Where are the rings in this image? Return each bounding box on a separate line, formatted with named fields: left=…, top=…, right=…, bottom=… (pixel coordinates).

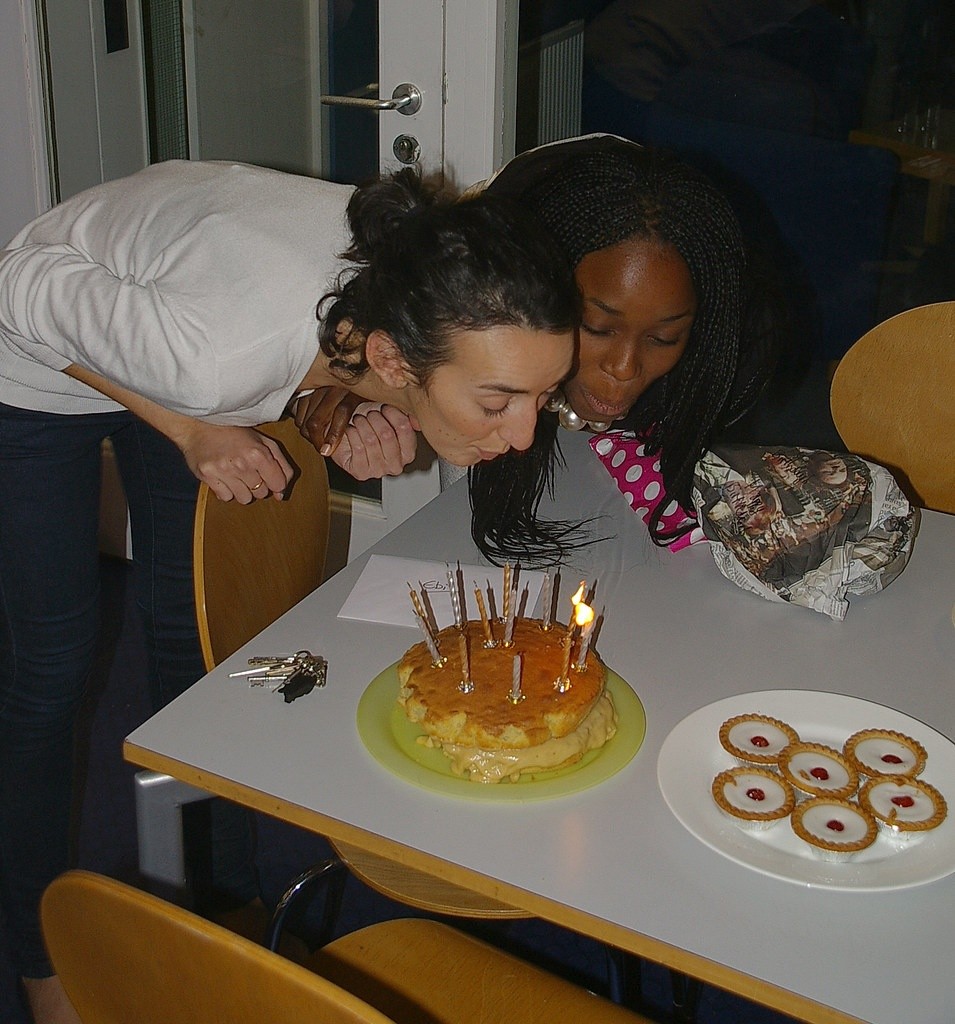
left=251, top=479, right=263, bottom=490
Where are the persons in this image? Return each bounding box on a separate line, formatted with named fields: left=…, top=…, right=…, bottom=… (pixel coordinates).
left=0, top=157, right=580, bottom=1023
left=294, top=133, right=747, bottom=570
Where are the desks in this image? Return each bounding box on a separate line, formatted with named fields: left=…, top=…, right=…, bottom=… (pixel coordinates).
left=848, top=107, right=955, bottom=246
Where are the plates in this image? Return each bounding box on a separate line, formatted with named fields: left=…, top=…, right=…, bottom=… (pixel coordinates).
left=355, top=659, right=646, bottom=802
left=656, top=689, right=955, bottom=890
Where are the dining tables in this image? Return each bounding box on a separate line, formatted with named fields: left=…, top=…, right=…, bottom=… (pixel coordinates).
left=117, top=424, right=954, bottom=1023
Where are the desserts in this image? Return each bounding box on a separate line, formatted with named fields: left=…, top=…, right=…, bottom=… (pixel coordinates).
left=710, top=712, right=947, bottom=862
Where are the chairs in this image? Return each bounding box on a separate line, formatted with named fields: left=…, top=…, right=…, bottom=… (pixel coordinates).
left=592, top=4, right=898, bottom=356
left=192, top=416, right=624, bottom=1006
left=828, top=302, right=955, bottom=515
left=40, top=869, right=657, bottom=1024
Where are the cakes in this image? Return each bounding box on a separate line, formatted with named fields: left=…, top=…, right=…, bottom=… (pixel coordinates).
left=397, top=616, right=619, bottom=783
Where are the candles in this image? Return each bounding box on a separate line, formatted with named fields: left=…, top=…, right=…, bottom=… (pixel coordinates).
left=406, top=562, right=606, bottom=705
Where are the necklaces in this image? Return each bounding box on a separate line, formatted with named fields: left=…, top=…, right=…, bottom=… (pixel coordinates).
left=545, top=389, right=628, bottom=431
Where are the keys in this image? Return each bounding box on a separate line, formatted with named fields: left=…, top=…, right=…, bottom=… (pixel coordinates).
left=230, top=650, right=328, bottom=704
